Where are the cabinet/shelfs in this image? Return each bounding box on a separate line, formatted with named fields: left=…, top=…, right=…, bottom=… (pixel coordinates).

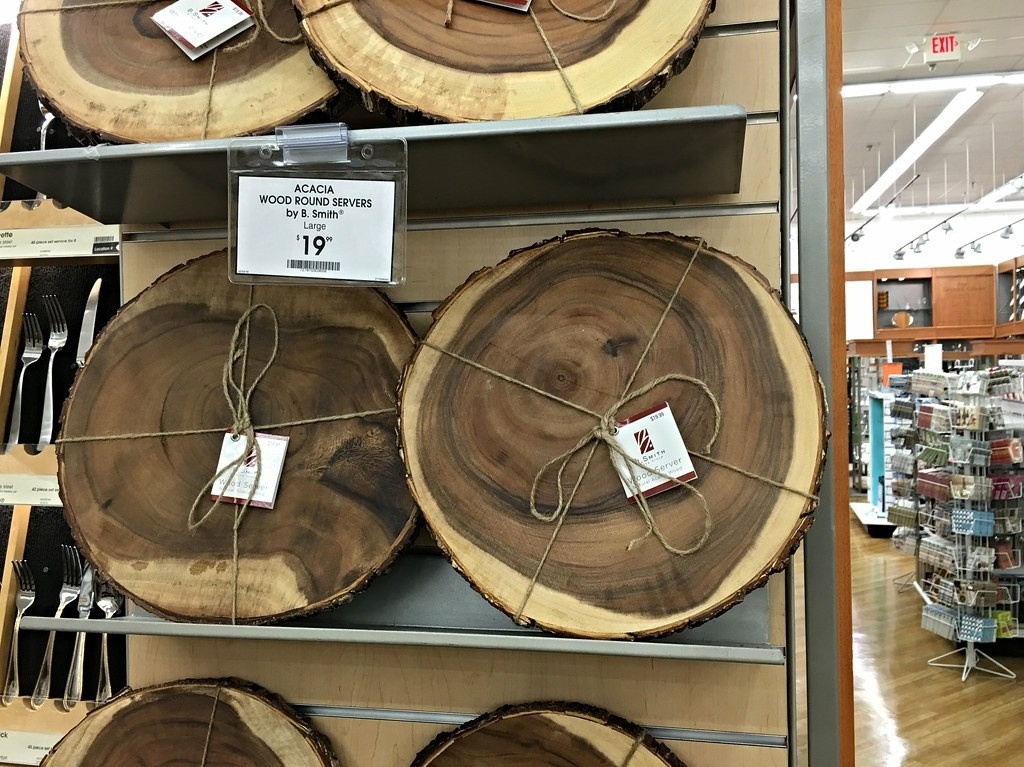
left=0, top=0, right=856, bottom=767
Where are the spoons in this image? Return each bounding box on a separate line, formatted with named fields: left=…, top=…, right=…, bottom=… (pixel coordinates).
left=94, top=573, right=124, bottom=708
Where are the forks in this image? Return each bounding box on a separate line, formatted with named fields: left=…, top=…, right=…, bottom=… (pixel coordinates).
left=35, top=295, right=68, bottom=452
left=2, top=559, right=36, bottom=706
left=4, top=312, right=43, bottom=455
left=30, top=544, right=83, bottom=710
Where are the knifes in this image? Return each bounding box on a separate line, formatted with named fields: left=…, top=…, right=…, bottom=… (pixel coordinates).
left=76, top=277, right=102, bottom=369
left=62, top=557, right=95, bottom=712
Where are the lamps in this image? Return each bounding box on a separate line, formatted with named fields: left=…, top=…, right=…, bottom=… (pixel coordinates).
left=850, top=221, right=1014, bottom=259
